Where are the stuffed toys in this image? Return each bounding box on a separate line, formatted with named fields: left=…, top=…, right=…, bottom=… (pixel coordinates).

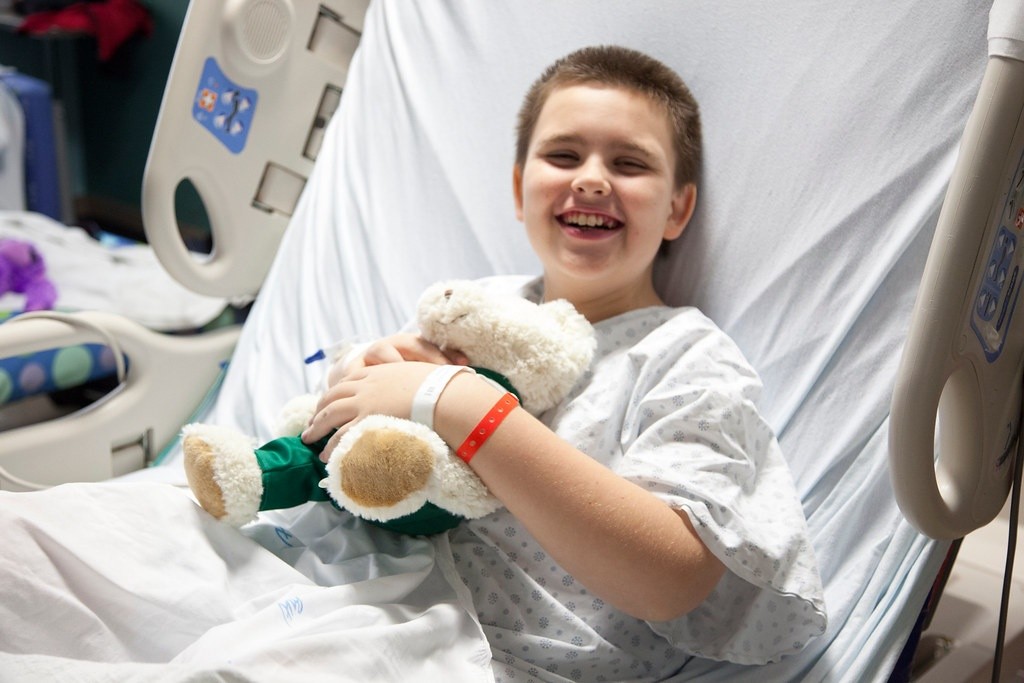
left=179, top=274, right=598, bottom=527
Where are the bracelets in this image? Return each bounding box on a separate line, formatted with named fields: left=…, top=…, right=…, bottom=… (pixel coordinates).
left=455, top=391, right=520, bottom=467
left=409, top=364, right=477, bottom=432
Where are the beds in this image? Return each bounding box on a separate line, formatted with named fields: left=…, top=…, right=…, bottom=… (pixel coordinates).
left=0, top=0, right=1024, bottom=683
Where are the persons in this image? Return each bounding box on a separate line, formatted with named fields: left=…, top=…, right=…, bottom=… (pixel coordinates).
left=300, top=44, right=828, bottom=683
